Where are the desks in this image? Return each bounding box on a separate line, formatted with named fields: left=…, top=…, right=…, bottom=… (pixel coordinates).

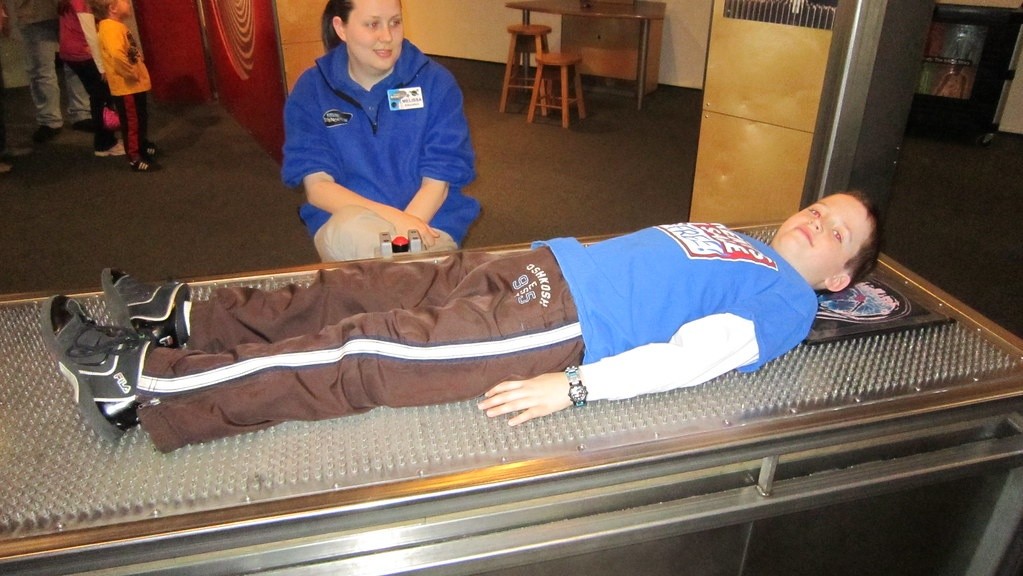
left=506, top=0, right=666, bottom=112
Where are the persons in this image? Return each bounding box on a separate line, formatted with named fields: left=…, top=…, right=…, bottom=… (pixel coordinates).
left=41, top=187, right=885, bottom=451
left=0, top=0, right=93, bottom=173
left=279, top=0, right=484, bottom=264
left=90, top=0, right=171, bottom=170
left=57, top=0, right=127, bottom=156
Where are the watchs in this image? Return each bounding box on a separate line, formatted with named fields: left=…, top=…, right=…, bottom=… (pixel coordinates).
left=563, top=366, right=589, bottom=408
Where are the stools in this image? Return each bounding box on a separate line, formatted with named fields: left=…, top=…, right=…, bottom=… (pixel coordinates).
left=527, top=53, right=586, bottom=128
left=500, top=25, right=552, bottom=116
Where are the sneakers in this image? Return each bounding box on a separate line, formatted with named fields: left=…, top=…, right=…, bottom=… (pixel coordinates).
left=41, top=294, right=156, bottom=441
left=94, top=140, right=126, bottom=156
left=102, top=266, right=190, bottom=348
left=141, top=143, right=167, bottom=157
left=130, top=158, right=161, bottom=172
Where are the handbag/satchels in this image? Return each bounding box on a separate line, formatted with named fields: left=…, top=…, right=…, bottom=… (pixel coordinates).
left=103, top=104, right=120, bottom=132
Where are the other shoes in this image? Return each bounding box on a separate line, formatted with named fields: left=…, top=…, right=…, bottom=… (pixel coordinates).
left=0, top=161, right=13, bottom=173
left=73, top=118, right=95, bottom=132
left=32, top=125, right=62, bottom=141
